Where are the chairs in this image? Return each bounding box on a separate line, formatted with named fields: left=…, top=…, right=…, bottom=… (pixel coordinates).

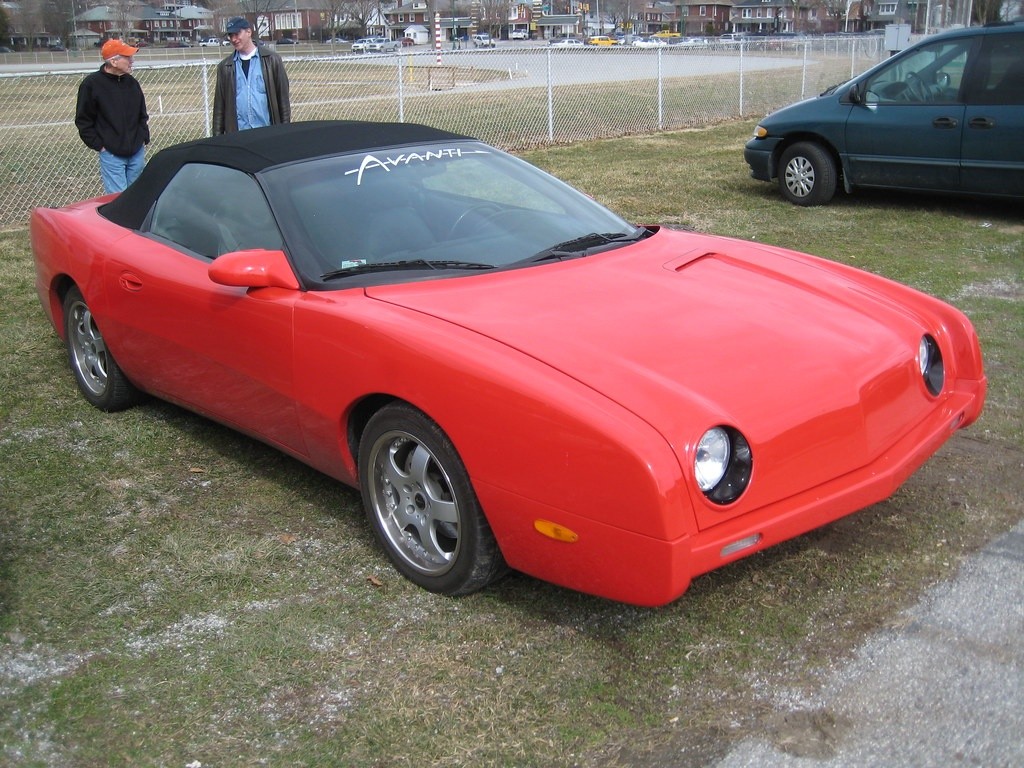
left=979, top=58, right=1024, bottom=104
left=219, top=192, right=285, bottom=255
left=360, top=175, right=437, bottom=262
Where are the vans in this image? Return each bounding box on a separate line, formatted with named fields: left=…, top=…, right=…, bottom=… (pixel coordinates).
left=717, top=33, right=740, bottom=44
left=473, top=35, right=478, bottom=42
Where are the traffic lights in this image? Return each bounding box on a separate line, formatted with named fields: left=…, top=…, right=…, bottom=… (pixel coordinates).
left=567, top=6, right=570, bottom=14
left=586, top=3, right=590, bottom=12
left=572, top=6, right=576, bottom=13
left=579, top=2, right=583, bottom=10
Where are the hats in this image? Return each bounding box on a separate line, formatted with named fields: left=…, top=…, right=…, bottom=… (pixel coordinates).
left=102, top=40, right=139, bottom=60
left=222, top=17, right=249, bottom=38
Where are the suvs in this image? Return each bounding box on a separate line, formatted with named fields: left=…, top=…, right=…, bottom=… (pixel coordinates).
left=512, top=28, right=529, bottom=40
left=589, top=35, right=619, bottom=47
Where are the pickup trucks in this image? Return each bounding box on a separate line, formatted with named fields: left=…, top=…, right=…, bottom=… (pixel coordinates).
left=551, top=39, right=585, bottom=50
left=654, top=30, right=682, bottom=38
left=367, top=38, right=397, bottom=52
left=198, top=38, right=228, bottom=48
left=548, top=33, right=584, bottom=42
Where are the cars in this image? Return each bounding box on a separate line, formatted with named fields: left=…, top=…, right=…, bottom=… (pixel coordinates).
left=130, top=41, right=149, bottom=47
left=168, top=41, right=193, bottom=48
left=867, top=29, right=887, bottom=36
left=632, top=38, right=667, bottom=47
left=254, top=39, right=266, bottom=48
left=222, top=39, right=230, bottom=46
left=327, top=37, right=347, bottom=45
left=368, top=37, right=376, bottom=43
left=276, top=38, right=299, bottom=45
left=397, top=37, right=413, bottom=46
left=745, top=23, right=1024, bottom=209
left=0, top=46, right=15, bottom=53
left=50, top=44, right=66, bottom=51
left=33, top=113, right=990, bottom=607
left=352, top=39, right=368, bottom=53
left=476, top=36, right=496, bottom=48
left=94, top=39, right=105, bottom=48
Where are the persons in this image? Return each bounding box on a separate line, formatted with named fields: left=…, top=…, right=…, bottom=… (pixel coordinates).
left=75, top=40, right=150, bottom=193
left=212, top=17, right=290, bottom=136
